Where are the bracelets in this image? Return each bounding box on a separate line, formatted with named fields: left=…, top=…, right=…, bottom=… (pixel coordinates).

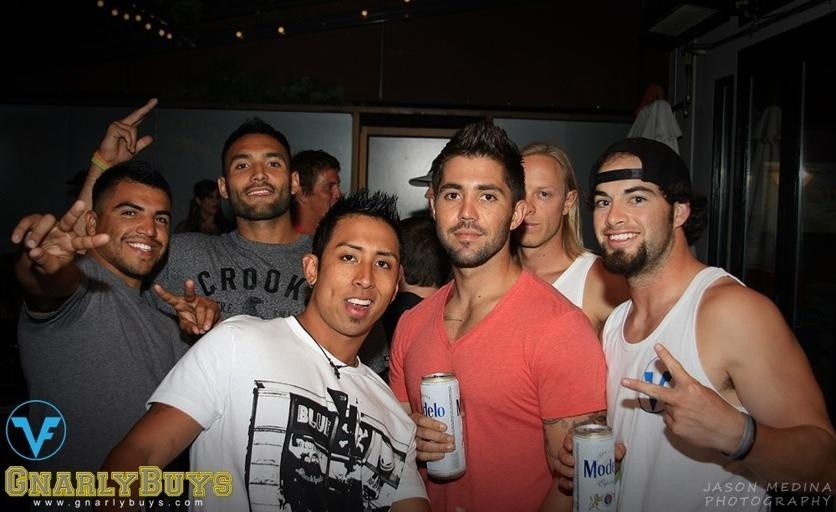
left=89, top=150, right=118, bottom=171
left=720, top=412, right=757, bottom=463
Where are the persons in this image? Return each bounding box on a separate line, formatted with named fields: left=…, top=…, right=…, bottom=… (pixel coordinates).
left=510, top=142, right=631, bottom=339
left=8, top=159, right=224, bottom=511
left=286, top=149, right=344, bottom=239
left=89, top=188, right=434, bottom=512
left=387, top=118, right=609, bottom=512
left=550, top=136, right=835, bottom=512
left=71, top=96, right=315, bottom=321
left=408, top=156, right=437, bottom=220
left=174, top=179, right=232, bottom=235
left=356, top=209, right=451, bottom=388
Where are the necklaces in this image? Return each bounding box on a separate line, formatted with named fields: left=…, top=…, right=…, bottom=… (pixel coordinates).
left=288, top=314, right=362, bottom=380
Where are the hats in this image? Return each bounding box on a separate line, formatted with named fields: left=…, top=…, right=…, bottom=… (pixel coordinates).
left=408, top=171, right=434, bottom=186
left=589, top=136, right=696, bottom=203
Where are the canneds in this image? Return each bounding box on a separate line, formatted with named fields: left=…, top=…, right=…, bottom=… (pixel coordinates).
left=573, top=425, right=616, bottom=512
left=419, top=373, right=467, bottom=483
